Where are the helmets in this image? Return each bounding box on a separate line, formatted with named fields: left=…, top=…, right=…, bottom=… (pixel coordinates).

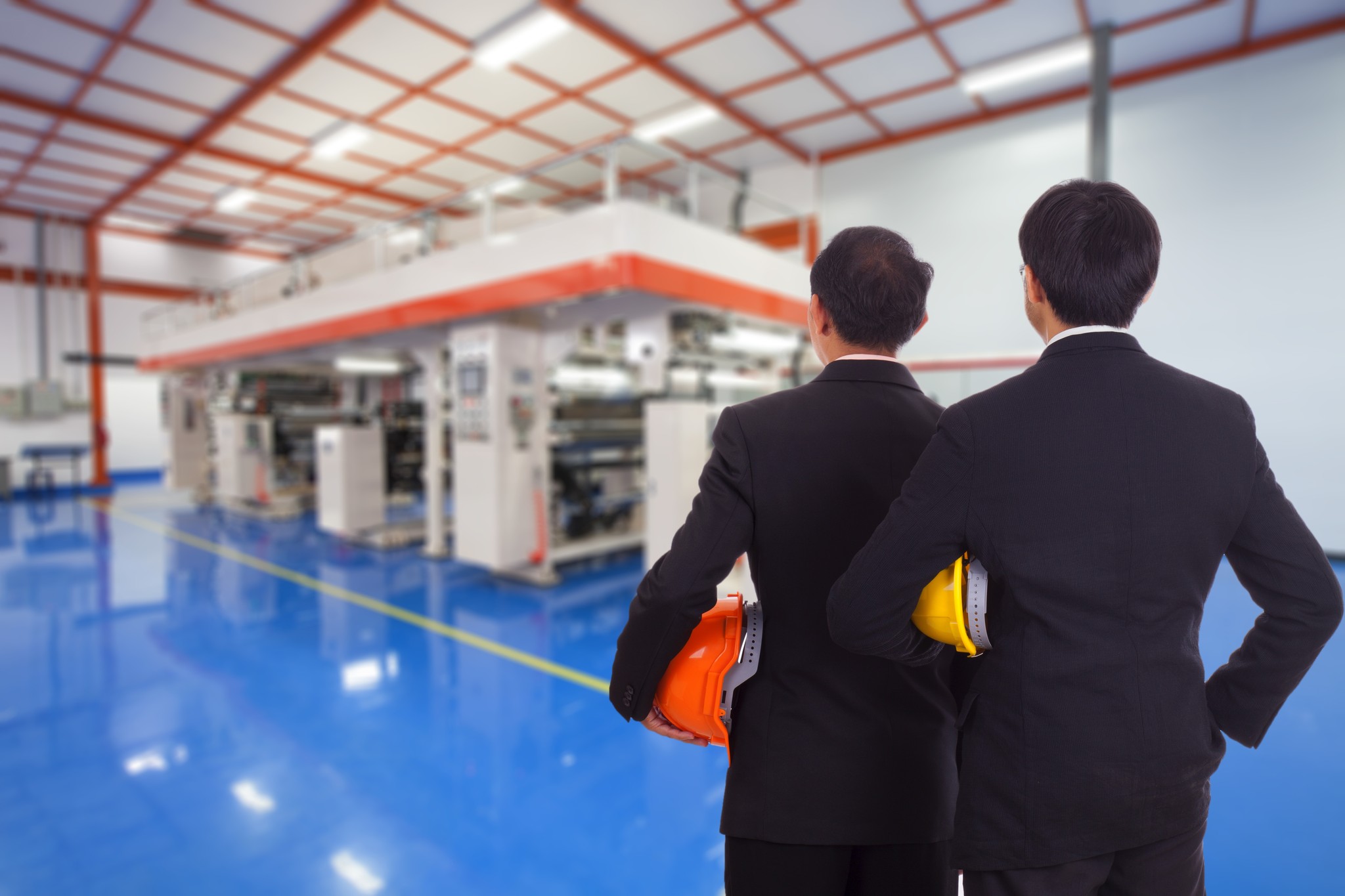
left=651, top=591, right=764, bottom=768
left=910, top=550, right=993, bottom=658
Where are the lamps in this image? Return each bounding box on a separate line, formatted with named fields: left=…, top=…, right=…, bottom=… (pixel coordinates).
left=469, top=4, right=570, bottom=74
left=629, top=103, right=715, bottom=145
left=961, top=30, right=1092, bottom=92
left=209, top=187, right=253, bottom=212
left=309, top=117, right=370, bottom=162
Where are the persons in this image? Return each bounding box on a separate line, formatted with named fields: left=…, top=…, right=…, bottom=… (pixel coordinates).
left=818, top=177, right=1345, bottom=896
left=607, top=223, right=963, bottom=896
